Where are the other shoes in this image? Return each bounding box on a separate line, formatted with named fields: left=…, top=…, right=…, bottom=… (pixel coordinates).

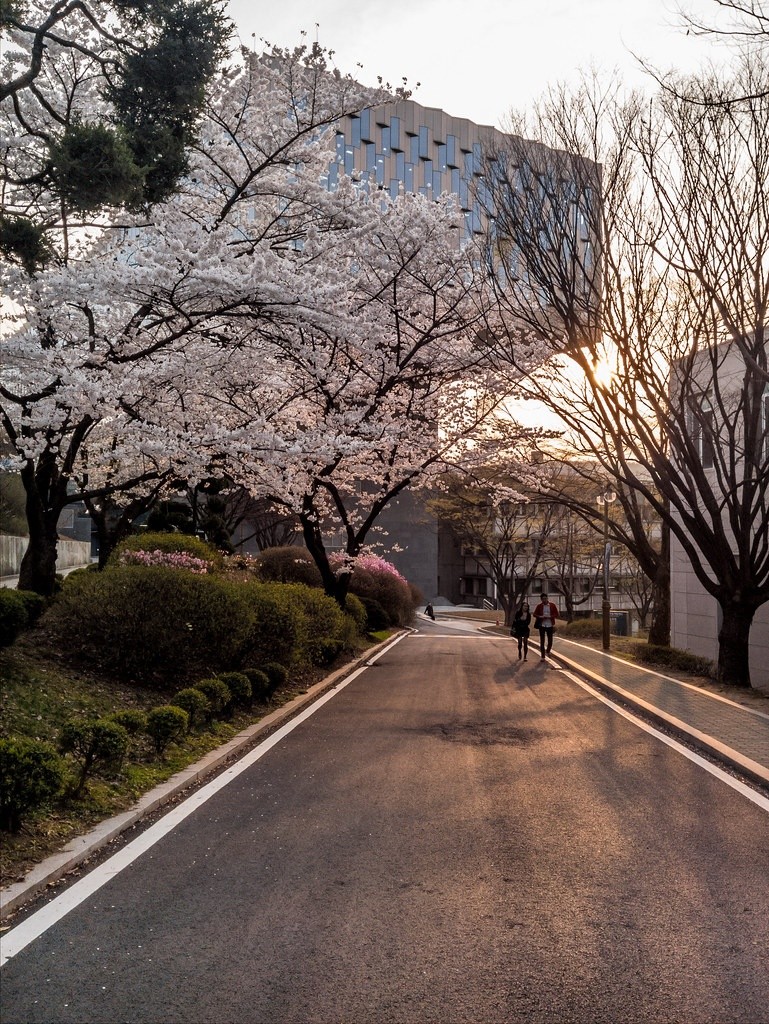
left=518, top=652, right=522, bottom=659
left=541, top=652, right=545, bottom=658
left=524, top=655, right=528, bottom=661
left=546, top=649, right=553, bottom=659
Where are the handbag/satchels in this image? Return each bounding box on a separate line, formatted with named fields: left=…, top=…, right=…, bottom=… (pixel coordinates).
left=510, top=611, right=521, bottom=638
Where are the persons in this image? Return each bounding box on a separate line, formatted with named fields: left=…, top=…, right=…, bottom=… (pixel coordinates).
left=514, top=602, right=531, bottom=661
left=424, top=601, right=435, bottom=620
left=533, top=593, right=558, bottom=662
